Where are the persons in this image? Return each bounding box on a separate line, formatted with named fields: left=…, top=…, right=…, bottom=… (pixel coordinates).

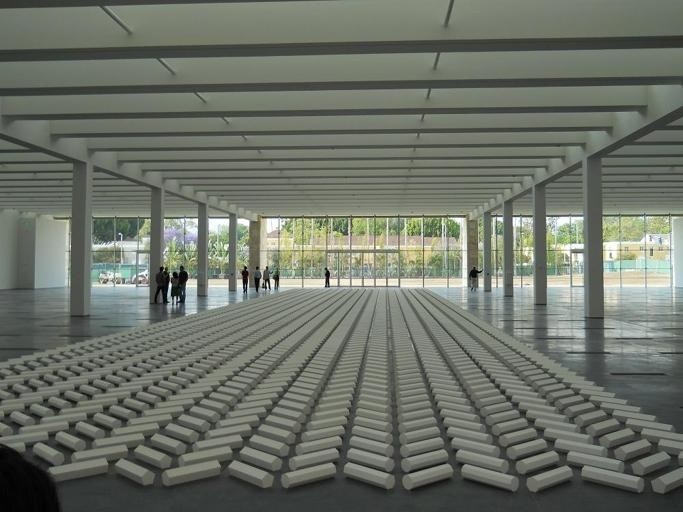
left=272, top=267, right=280, bottom=290
left=240, top=266, right=249, bottom=294
left=153, top=266, right=166, bottom=305
left=170, top=271, right=181, bottom=305
left=176, top=264, right=188, bottom=304
left=0, top=443, right=62, bottom=512
left=323, top=267, right=331, bottom=288
left=163, top=266, right=170, bottom=303
left=467, top=266, right=483, bottom=292
left=262, top=265, right=273, bottom=290
left=252, top=266, right=262, bottom=292
